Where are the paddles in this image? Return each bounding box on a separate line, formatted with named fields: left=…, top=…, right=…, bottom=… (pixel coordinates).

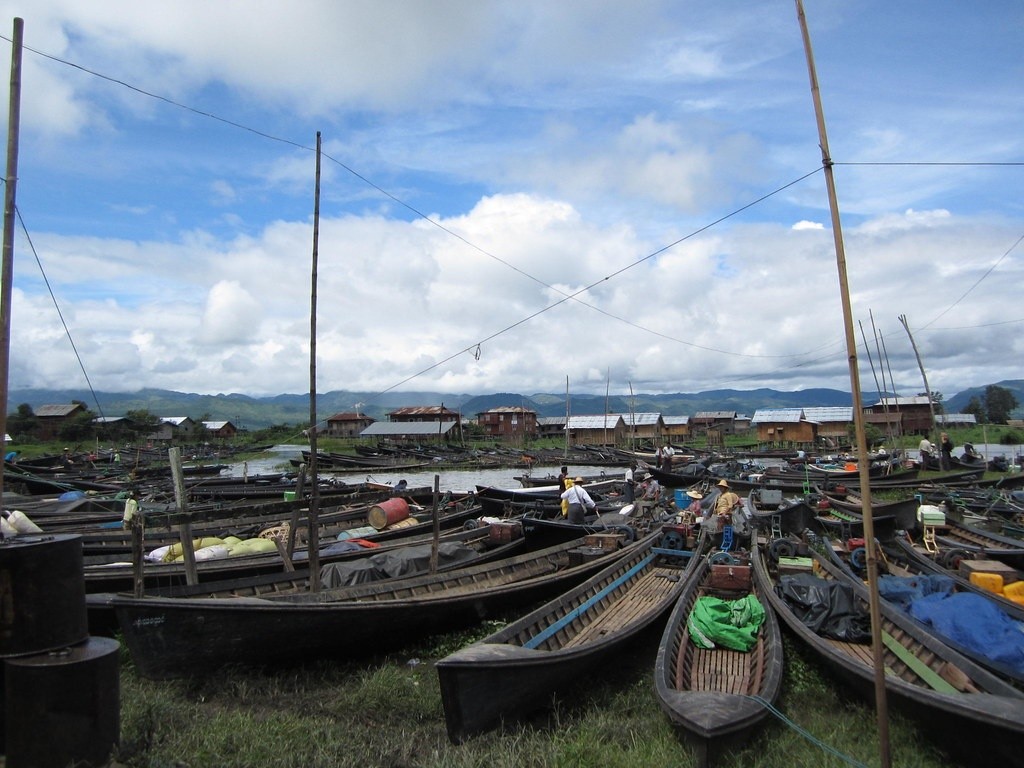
left=940, top=660, right=982, bottom=694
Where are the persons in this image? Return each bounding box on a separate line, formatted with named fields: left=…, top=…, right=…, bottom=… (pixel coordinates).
left=655, top=441, right=675, bottom=473
left=797, top=448, right=806, bottom=457
left=625, top=465, right=637, bottom=482
left=87, top=451, right=96, bottom=461
left=838, top=432, right=977, bottom=471
left=114, top=451, right=121, bottom=463
left=713, top=480, right=733, bottom=549
left=687, top=491, right=702, bottom=517
left=59, top=448, right=69, bottom=465
left=558, top=467, right=598, bottom=524
left=641, top=473, right=660, bottom=501
left=393, top=479, right=419, bottom=506
left=4, top=450, right=22, bottom=464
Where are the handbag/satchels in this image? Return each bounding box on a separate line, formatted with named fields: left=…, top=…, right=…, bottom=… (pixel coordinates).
left=581, top=503, right=587, bottom=514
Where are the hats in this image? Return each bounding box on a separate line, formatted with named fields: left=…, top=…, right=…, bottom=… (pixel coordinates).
left=715, top=480, right=730, bottom=488
left=643, top=473, right=654, bottom=480
left=686, top=490, right=702, bottom=498
left=573, top=477, right=583, bottom=483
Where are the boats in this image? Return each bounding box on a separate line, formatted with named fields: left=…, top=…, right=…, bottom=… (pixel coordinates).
left=3, top=437, right=1023, bottom=768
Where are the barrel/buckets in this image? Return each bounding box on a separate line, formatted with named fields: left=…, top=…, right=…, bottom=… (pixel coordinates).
left=0, top=636, right=122, bottom=768
left=337, top=498, right=419, bottom=541
left=0, top=531, right=89, bottom=658
left=674, top=489, right=692, bottom=510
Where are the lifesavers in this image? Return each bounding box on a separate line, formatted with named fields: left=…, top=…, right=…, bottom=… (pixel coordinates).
left=617, top=525, right=634, bottom=547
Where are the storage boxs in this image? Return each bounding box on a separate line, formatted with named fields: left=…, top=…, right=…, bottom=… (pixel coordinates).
left=748, top=473, right=764, bottom=483
left=778, top=555, right=812, bottom=580
left=490, top=522, right=521, bottom=546
left=712, top=564, right=752, bottom=589
left=585, top=533, right=625, bottom=553
left=760, top=489, right=781, bottom=504
left=921, top=511, right=945, bottom=525
left=958, top=560, right=1016, bottom=586
